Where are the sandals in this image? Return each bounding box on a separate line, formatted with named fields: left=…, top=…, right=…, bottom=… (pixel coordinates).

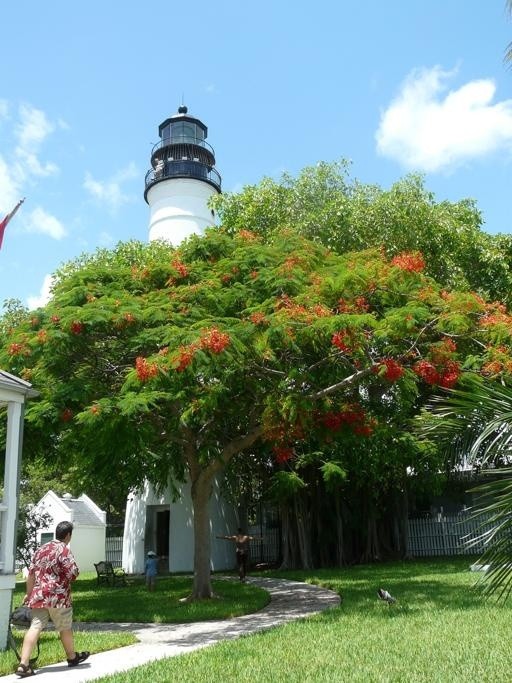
left=67, top=651, right=90, bottom=667
left=16, top=664, right=34, bottom=676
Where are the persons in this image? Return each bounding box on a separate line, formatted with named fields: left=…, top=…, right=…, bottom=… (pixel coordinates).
left=215, top=528, right=266, bottom=582
left=143, top=550, right=162, bottom=594
left=14, top=520, right=89, bottom=677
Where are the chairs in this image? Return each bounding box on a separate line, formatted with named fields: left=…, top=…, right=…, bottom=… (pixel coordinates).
left=93, top=561, right=128, bottom=586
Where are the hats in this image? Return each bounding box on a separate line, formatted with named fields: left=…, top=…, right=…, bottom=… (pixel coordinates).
left=148, top=551, right=156, bottom=556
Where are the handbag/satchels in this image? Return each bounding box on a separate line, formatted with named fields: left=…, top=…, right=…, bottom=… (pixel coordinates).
left=12, top=603, right=31, bottom=627
